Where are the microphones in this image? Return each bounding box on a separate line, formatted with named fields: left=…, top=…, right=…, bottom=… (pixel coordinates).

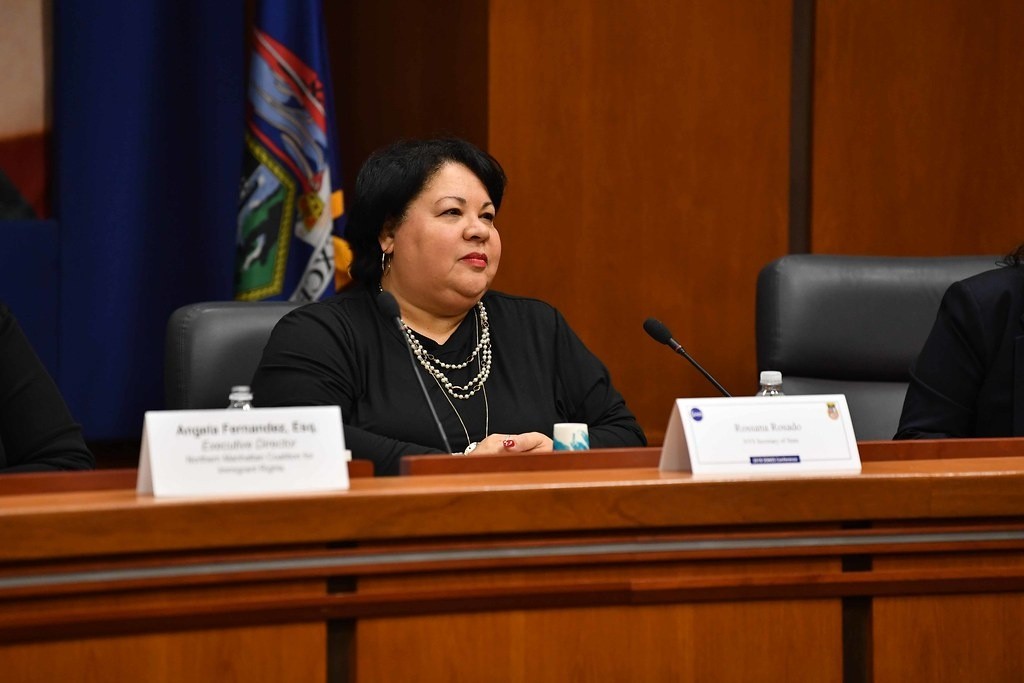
left=643, top=318, right=732, bottom=397
left=375, top=291, right=452, bottom=456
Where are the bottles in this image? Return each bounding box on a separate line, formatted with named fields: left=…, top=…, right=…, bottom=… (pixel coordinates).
left=756, top=370, right=787, bottom=396
left=226, top=385, right=254, bottom=409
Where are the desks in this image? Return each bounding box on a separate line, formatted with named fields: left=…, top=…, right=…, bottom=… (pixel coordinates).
left=0, top=438, right=1024, bottom=683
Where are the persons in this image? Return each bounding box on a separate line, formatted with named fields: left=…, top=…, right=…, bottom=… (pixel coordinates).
left=251, top=138, right=648, bottom=476
left=893, top=244, right=1024, bottom=439
left=0, top=302, right=97, bottom=474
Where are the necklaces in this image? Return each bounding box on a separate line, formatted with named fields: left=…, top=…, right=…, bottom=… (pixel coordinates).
left=396, top=300, right=492, bottom=452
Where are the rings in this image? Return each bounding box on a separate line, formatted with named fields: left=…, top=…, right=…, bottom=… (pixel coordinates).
left=505, top=435, right=509, bottom=440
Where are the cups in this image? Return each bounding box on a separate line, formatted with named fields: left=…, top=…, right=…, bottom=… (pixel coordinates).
left=553, top=422, right=589, bottom=451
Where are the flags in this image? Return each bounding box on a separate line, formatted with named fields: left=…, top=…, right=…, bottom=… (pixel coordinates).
left=53, top=0, right=353, bottom=441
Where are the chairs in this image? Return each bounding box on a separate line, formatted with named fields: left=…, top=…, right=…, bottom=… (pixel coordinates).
left=754, top=253, right=1022, bottom=440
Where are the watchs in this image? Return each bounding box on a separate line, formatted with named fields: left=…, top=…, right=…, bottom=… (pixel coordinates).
left=463, top=442, right=477, bottom=456
